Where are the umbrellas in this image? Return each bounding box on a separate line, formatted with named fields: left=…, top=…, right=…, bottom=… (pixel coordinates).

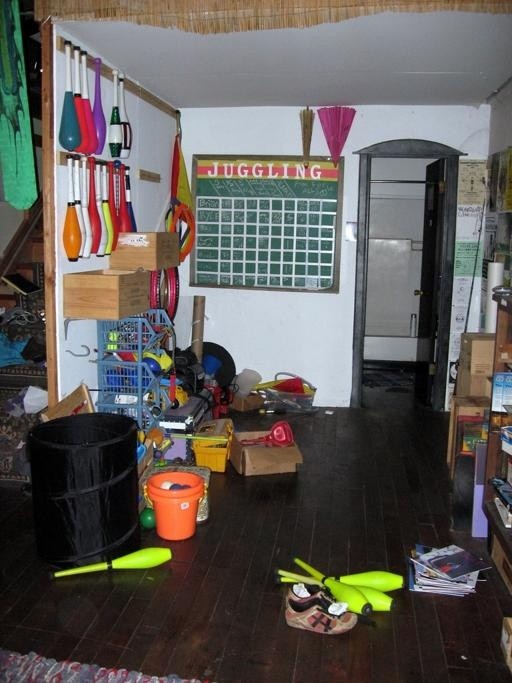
left=316, top=105, right=356, bottom=167
left=298, top=104, right=315, bottom=168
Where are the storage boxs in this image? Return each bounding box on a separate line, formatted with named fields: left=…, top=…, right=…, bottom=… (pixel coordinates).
left=499, top=615, right=512, bottom=674
left=453, top=364, right=493, bottom=399
left=459, top=332, right=496, bottom=373
left=193, top=417, right=234, bottom=472
left=109, top=230, right=182, bottom=272
left=264, top=387, right=317, bottom=413
left=229, top=392, right=264, bottom=412
left=63, top=268, right=151, bottom=323
left=230, top=429, right=304, bottom=476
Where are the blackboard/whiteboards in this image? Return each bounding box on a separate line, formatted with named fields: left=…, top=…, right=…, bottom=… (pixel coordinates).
left=189, top=151, right=346, bottom=296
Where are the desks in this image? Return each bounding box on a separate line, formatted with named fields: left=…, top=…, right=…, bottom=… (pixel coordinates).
left=446, top=392, right=491, bottom=480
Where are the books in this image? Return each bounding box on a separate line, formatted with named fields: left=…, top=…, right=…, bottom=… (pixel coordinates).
left=405, top=543, right=493, bottom=597
left=487, top=425, right=511, bottom=530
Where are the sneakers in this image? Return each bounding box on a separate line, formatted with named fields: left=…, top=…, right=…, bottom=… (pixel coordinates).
left=286, top=582, right=336, bottom=604
left=286, top=596, right=358, bottom=635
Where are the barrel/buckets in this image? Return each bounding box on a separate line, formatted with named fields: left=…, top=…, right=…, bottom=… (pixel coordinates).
left=142, top=468, right=210, bottom=544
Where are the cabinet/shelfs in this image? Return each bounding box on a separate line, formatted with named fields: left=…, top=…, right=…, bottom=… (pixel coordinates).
left=481, top=290, right=512, bottom=597
left=97, top=308, right=174, bottom=461
